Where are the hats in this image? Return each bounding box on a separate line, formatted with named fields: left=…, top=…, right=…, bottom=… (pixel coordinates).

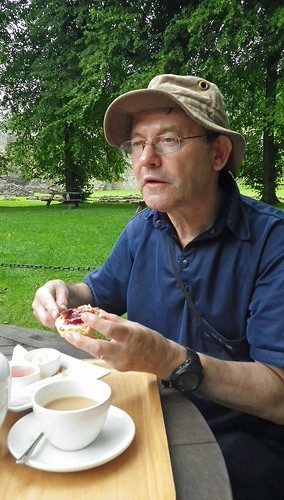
left=103, top=73, right=246, bottom=180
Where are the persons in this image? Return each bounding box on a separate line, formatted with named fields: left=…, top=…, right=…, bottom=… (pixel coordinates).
left=31, top=74, right=284, bottom=500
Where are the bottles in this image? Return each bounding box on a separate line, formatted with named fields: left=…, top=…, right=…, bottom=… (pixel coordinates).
left=0, top=353, right=10, bottom=425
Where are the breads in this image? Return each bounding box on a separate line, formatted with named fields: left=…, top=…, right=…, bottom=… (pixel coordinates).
left=55, top=304, right=105, bottom=338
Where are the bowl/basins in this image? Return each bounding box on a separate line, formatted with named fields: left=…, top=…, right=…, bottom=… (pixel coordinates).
left=28, top=348, right=61, bottom=378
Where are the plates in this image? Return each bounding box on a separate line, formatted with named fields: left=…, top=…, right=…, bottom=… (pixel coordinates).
left=7, top=405, right=136, bottom=472
left=8, top=348, right=111, bottom=412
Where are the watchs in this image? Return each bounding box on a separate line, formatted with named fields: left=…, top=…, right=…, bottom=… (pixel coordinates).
left=161, top=345, right=204, bottom=394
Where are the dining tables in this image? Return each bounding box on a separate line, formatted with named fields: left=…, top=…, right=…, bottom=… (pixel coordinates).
left=0, top=323, right=234, bottom=500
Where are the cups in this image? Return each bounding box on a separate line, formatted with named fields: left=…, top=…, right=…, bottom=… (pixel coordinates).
left=33, top=379, right=111, bottom=451
left=9, top=361, right=40, bottom=405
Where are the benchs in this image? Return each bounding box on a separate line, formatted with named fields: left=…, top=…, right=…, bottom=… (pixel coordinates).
left=131, top=200, right=146, bottom=214
left=42, top=191, right=82, bottom=209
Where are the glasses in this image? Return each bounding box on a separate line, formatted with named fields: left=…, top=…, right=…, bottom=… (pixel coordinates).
left=120, top=133, right=209, bottom=158
